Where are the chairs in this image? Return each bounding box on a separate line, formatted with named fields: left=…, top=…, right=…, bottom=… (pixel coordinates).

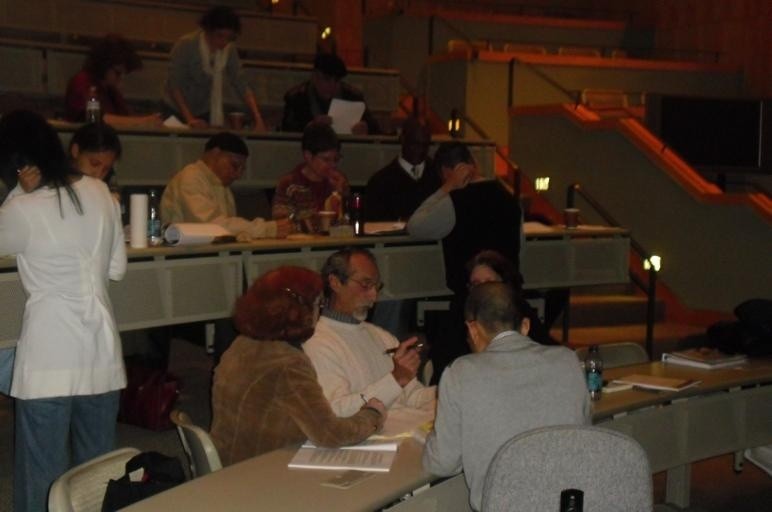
left=481, top=423, right=654, bottom=512
left=169, top=408, right=223, bottom=480
left=575, top=343, right=649, bottom=368
left=48, top=446, right=144, bottom=512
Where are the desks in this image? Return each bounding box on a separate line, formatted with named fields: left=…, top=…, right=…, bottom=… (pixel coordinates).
left=0, top=224, right=631, bottom=345
left=44, top=119, right=498, bottom=187
left=115, top=356, right=772, bottom=512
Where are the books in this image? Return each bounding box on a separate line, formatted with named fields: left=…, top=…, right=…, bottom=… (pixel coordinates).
left=612, top=373, right=701, bottom=391
left=288, top=439, right=403, bottom=473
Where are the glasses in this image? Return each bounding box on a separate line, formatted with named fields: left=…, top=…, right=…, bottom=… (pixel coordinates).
left=349, top=276, right=385, bottom=292
left=311, top=153, right=346, bottom=165
left=465, top=280, right=481, bottom=289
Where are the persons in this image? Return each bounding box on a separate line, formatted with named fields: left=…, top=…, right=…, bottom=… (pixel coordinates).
left=0, top=109, right=130, bottom=507
left=165, top=6, right=266, bottom=131
left=211, top=265, right=389, bottom=467
left=422, top=278, right=596, bottom=512
left=155, top=131, right=298, bottom=241
left=449, top=245, right=561, bottom=368
left=363, top=112, right=442, bottom=226
left=284, top=54, right=379, bottom=135
left=407, top=141, right=525, bottom=350
left=301, top=246, right=443, bottom=422
left=64, top=35, right=167, bottom=131
left=273, top=123, right=352, bottom=228
left=65, top=121, right=124, bottom=182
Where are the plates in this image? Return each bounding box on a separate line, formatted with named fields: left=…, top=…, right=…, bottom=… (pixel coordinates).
left=364, top=221, right=407, bottom=235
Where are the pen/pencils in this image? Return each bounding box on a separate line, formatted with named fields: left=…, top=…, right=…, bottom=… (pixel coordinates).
left=382, top=343, right=423, bottom=354
left=360, top=394, right=370, bottom=404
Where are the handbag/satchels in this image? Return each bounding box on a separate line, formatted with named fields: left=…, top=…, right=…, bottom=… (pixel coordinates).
left=115, top=356, right=182, bottom=431
left=101, top=449, right=189, bottom=512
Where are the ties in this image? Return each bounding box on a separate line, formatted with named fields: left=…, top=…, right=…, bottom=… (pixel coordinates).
left=412, top=164, right=421, bottom=179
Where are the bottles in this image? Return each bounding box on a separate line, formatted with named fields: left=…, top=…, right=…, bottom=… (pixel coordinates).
left=585, top=343, right=603, bottom=402
left=350, top=192, right=364, bottom=236
left=559, top=489, right=582, bottom=512
left=149, top=190, right=163, bottom=246
left=85, top=85, right=100, bottom=126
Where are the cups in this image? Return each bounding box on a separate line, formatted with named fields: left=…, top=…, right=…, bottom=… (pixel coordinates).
left=318, top=211, right=336, bottom=235
left=564, top=208, right=580, bottom=230
